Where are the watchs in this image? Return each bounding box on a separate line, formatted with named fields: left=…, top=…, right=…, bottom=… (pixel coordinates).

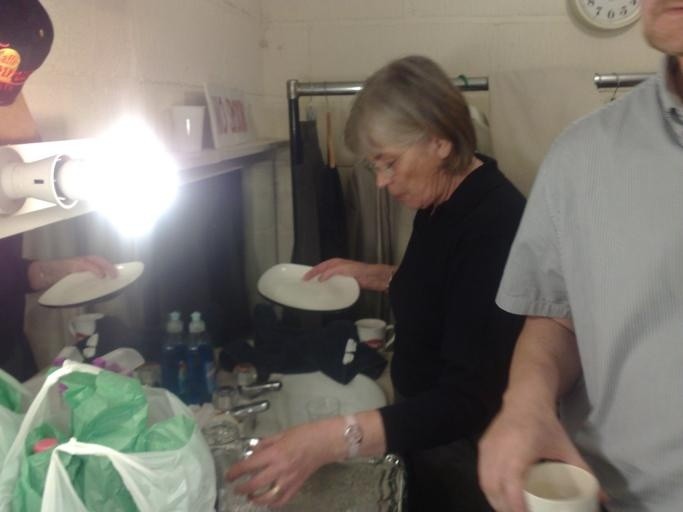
left=343, top=414, right=363, bottom=460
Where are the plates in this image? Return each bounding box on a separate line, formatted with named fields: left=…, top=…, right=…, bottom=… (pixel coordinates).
left=272, top=369, right=388, bottom=441
left=254, top=260, right=363, bottom=314
left=34, top=259, right=146, bottom=310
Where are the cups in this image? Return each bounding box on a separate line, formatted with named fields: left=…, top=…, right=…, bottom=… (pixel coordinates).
left=519, top=461, right=602, bottom=512
left=353, top=317, right=396, bottom=354
left=66, top=312, right=105, bottom=344
left=303, top=395, right=342, bottom=421
left=170, top=101, right=207, bottom=156
left=219, top=434, right=274, bottom=512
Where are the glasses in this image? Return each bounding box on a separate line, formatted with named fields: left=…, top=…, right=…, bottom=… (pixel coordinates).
left=360, top=149, right=414, bottom=179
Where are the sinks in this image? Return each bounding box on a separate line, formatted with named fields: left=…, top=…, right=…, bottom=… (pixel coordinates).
left=248, top=365, right=388, bottom=447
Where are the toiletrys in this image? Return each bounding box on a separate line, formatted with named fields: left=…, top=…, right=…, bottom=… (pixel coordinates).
left=160, top=310, right=214, bottom=405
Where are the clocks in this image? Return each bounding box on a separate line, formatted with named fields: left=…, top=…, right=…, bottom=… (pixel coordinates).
left=571, top=0, right=641, bottom=30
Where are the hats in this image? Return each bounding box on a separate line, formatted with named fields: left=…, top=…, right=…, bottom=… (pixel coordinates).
left=0, top=1, right=53, bottom=108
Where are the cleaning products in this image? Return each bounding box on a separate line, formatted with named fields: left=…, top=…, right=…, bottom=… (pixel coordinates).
left=160, top=311, right=185, bottom=403
left=186, top=309, right=217, bottom=403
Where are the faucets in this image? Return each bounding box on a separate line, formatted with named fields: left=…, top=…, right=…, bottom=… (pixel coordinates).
left=216, top=399, right=270, bottom=422
left=207, top=380, right=284, bottom=413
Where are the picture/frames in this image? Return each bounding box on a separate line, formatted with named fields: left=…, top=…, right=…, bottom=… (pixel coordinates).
left=200, top=84, right=254, bottom=146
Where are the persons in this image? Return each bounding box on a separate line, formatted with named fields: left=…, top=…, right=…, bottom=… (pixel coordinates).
left=0, top=234, right=118, bottom=382
left=219, top=63, right=527, bottom=512
left=476, top=0, right=683, bottom=512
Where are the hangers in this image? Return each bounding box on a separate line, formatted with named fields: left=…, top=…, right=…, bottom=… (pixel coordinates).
left=610, top=71, right=621, bottom=102
left=301, top=79, right=336, bottom=173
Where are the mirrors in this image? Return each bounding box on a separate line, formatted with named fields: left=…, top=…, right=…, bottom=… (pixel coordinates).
left=1, top=161, right=276, bottom=467
